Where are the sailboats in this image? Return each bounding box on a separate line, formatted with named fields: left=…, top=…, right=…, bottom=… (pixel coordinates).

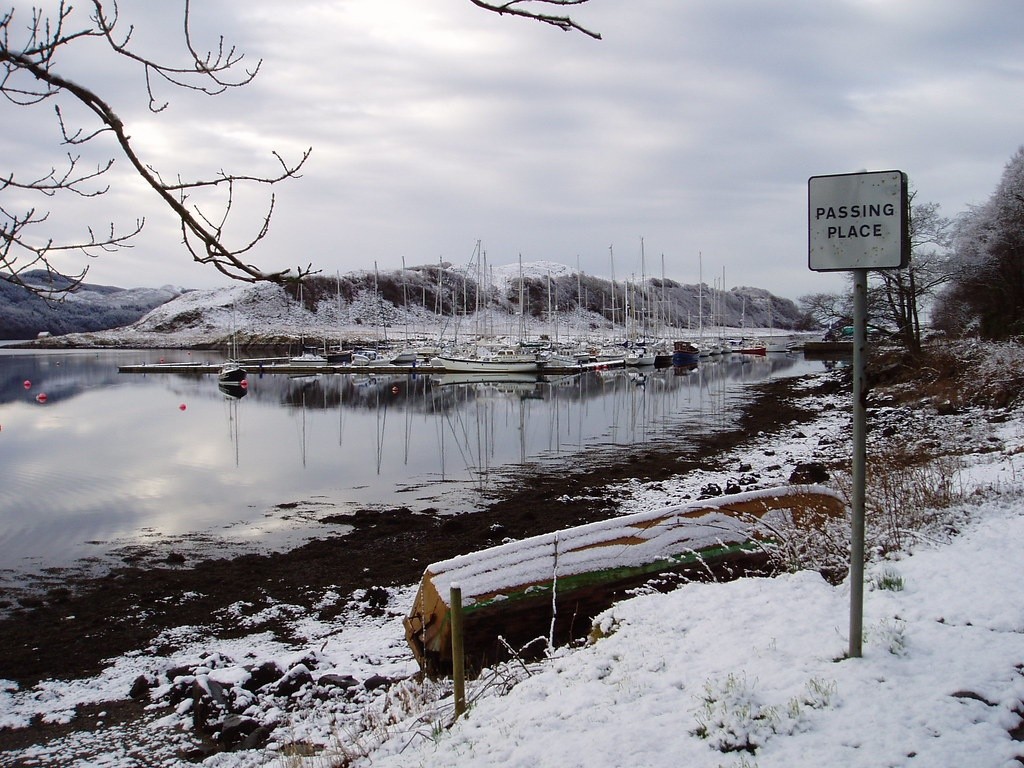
left=218, top=299, right=248, bottom=383
left=287, top=353, right=766, bottom=500
left=218, top=381, right=248, bottom=468
left=288, top=238, right=797, bottom=374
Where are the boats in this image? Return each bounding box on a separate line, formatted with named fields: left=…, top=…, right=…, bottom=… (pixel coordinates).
left=402, top=484, right=850, bottom=683
